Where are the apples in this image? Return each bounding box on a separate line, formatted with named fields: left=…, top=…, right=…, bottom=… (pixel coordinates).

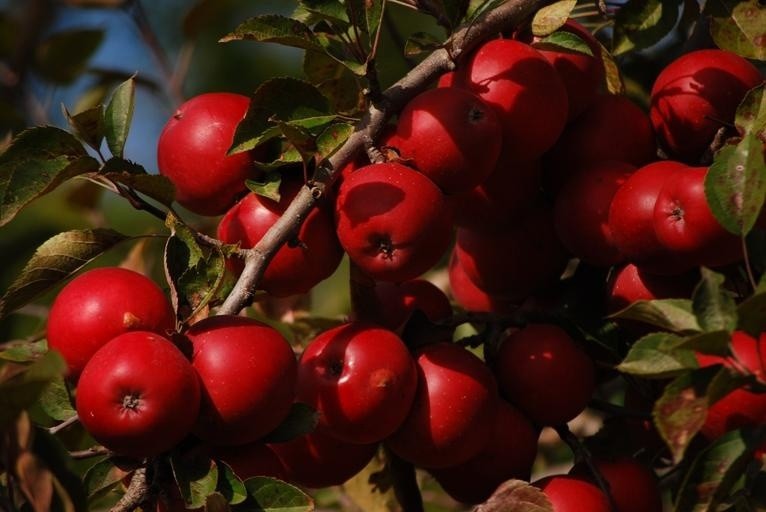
left=46, top=17, right=766, bottom=511
